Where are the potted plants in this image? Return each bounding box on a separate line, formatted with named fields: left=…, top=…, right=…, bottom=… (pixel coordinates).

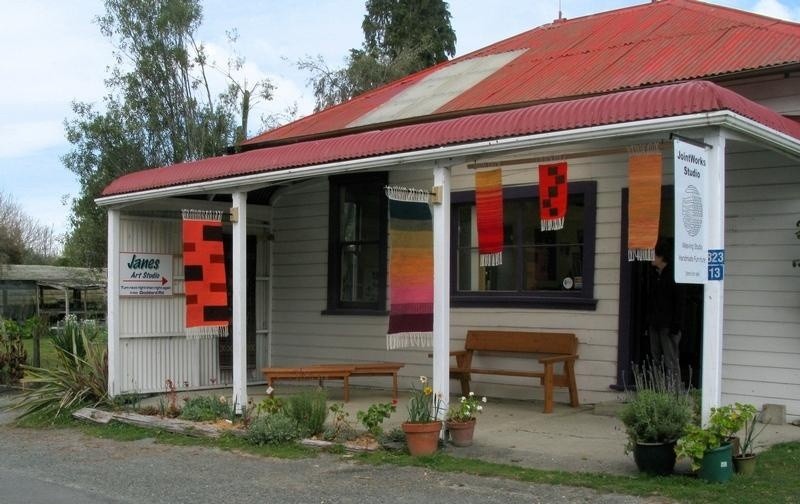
left=612, top=384, right=691, bottom=474
left=680, top=403, right=761, bottom=482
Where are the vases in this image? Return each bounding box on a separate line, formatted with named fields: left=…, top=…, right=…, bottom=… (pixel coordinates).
left=732, top=452, right=758, bottom=476
left=721, top=434, right=742, bottom=455
left=446, top=420, right=476, bottom=446
left=401, top=422, right=443, bottom=455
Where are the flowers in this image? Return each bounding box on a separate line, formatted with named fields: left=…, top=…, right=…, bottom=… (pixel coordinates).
left=454, top=388, right=491, bottom=420
left=403, top=375, right=445, bottom=422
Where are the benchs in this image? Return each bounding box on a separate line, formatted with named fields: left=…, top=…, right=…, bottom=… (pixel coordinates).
left=261, top=361, right=353, bottom=406
left=424, top=326, right=585, bottom=410
left=314, top=359, right=405, bottom=404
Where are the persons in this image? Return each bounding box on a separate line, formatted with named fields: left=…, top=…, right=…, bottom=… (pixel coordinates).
left=635, top=239, right=688, bottom=389
